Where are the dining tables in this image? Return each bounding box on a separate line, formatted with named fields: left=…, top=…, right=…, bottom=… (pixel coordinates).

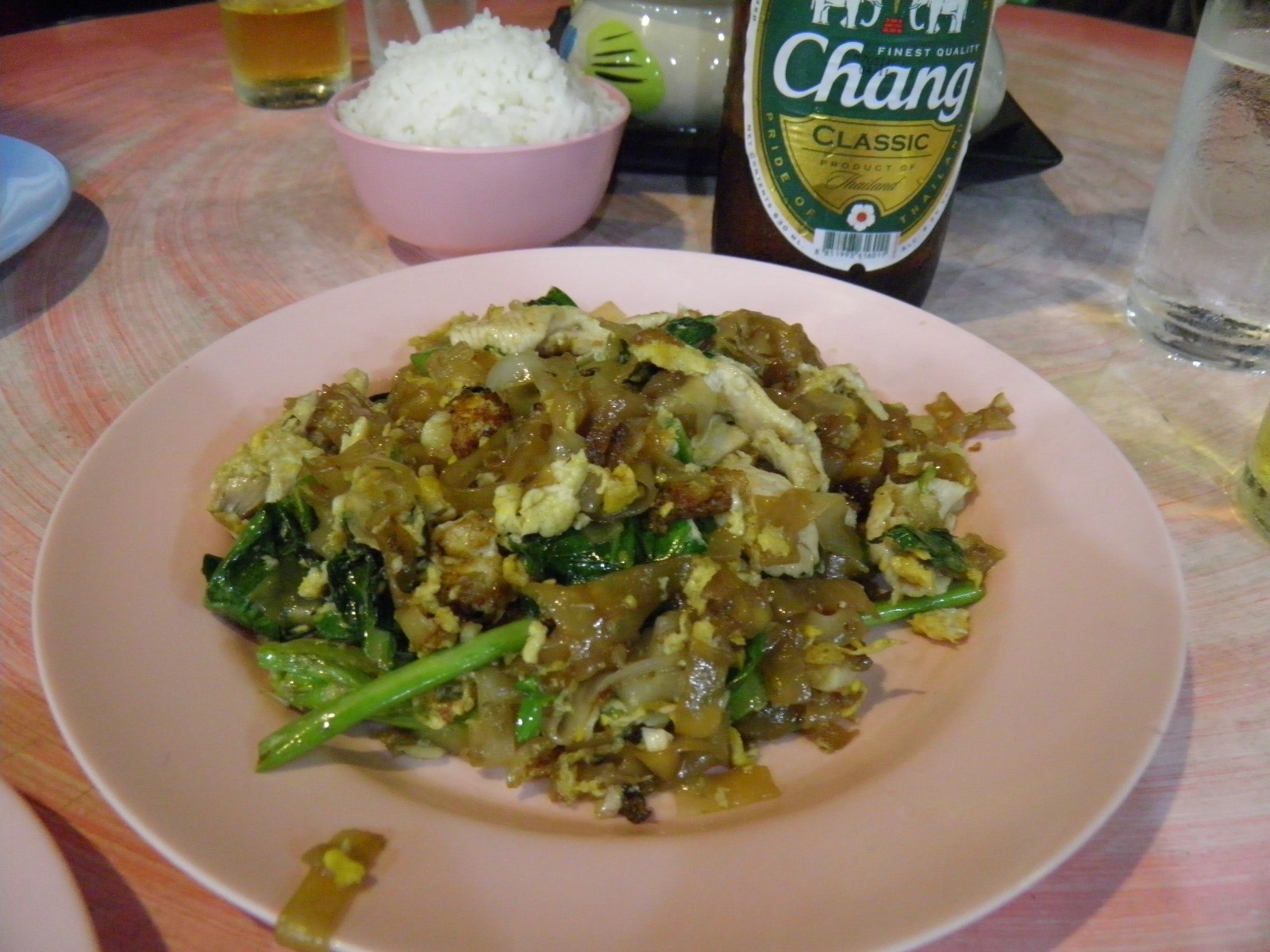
left=1, top=2, right=1266, bottom=951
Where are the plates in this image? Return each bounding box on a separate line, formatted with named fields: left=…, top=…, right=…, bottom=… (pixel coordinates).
left=34, top=248, right=1187, bottom=952
left=2, top=777, right=102, bottom=949
left=541, top=6, right=1063, bottom=182
left=0, top=134, right=71, bottom=264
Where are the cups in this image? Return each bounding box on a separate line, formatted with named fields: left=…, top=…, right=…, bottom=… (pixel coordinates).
left=1236, top=395, right=1269, bottom=540
left=217, top=0, right=351, bottom=108
left=363, top=0, right=476, bottom=73
left=971, top=1, right=1007, bottom=131
left=557, top=0, right=738, bottom=130
left=1128, top=0, right=1269, bottom=376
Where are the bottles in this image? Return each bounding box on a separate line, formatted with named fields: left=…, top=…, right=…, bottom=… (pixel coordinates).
left=712, top=1, right=993, bottom=308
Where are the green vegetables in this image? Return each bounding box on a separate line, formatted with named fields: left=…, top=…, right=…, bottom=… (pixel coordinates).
left=203, top=494, right=989, bottom=771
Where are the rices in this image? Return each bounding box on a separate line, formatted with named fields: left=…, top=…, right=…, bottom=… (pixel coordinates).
left=337, top=2, right=625, bottom=150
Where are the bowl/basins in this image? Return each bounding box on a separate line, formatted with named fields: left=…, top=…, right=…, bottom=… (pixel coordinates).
left=328, top=75, right=632, bottom=264
